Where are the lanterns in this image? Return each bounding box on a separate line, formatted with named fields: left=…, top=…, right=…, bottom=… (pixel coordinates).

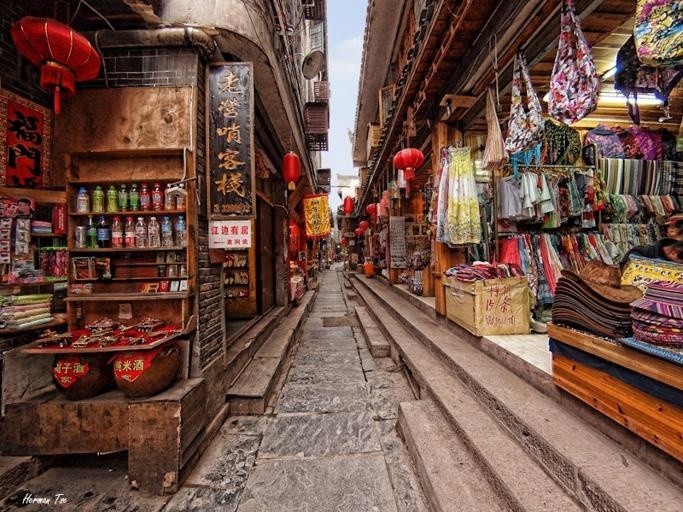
left=353, top=227, right=363, bottom=236
left=359, top=220, right=369, bottom=230
left=282, top=152, right=300, bottom=190
left=366, top=203, right=376, bottom=217
left=11, top=14, right=100, bottom=117
left=342, top=196, right=353, bottom=215
left=392, top=147, right=425, bottom=180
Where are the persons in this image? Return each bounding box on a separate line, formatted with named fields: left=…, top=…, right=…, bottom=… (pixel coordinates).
left=16, top=198, right=35, bottom=219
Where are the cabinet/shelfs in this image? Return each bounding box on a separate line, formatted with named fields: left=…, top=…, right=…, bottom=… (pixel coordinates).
left=63, top=211, right=187, bottom=301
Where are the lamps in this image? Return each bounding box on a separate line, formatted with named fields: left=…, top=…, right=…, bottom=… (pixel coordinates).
left=279, top=49, right=324, bottom=80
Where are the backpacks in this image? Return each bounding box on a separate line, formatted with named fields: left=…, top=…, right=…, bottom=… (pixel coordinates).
left=614, top=0, right=683, bottom=125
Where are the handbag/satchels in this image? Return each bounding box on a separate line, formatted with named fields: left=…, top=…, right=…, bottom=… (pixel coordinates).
left=547, top=48, right=599, bottom=125
left=505, top=107, right=544, bottom=153
left=480, top=131, right=510, bottom=171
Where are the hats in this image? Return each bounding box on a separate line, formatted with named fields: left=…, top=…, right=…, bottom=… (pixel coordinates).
left=551, top=258, right=683, bottom=348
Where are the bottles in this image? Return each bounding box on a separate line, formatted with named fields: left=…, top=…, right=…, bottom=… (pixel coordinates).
left=74, top=183, right=187, bottom=247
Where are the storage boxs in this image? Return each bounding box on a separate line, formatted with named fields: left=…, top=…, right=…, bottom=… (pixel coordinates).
left=38, top=246, right=68, bottom=277
